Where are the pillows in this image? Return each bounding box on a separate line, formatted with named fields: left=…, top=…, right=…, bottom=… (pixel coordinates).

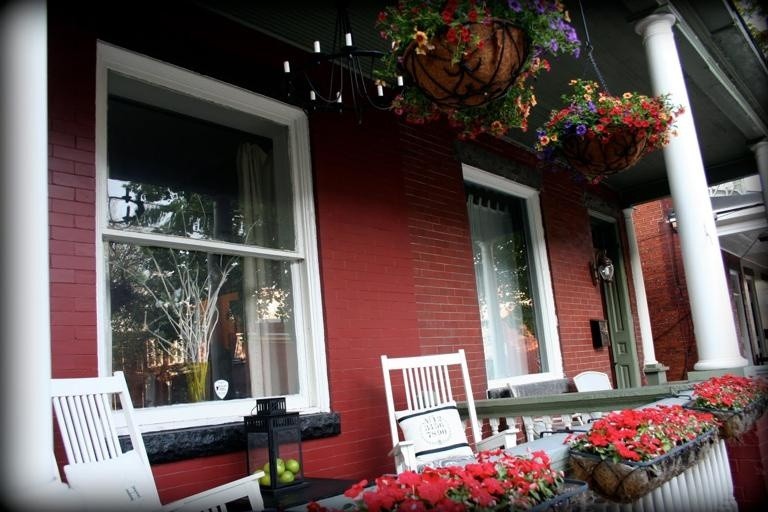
left=64, top=450, right=163, bottom=512
left=396, top=399, right=474, bottom=465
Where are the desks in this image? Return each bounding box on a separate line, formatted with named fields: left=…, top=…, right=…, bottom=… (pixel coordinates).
left=209, top=475, right=359, bottom=512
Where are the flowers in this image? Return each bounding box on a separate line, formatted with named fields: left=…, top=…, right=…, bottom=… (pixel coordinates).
left=562, top=404, right=722, bottom=464
left=374, top=0, right=581, bottom=139
left=306, top=446, right=566, bottom=512
left=688, top=370, right=768, bottom=413
left=530, top=76, right=685, bottom=183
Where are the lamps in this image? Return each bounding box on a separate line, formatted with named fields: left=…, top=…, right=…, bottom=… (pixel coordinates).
left=283, top=0, right=405, bottom=117
left=591, top=253, right=615, bottom=284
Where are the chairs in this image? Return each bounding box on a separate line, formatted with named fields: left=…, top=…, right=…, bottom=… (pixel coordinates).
left=573, top=370, right=626, bottom=423
left=52, top=371, right=265, bottom=512
left=506, top=383, right=586, bottom=441
left=380, top=348, right=519, bottom=488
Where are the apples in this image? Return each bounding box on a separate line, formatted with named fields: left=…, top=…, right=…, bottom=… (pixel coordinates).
left=250, top=457, right=300, bottom=486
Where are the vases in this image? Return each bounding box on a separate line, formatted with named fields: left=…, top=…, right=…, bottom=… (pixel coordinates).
left=683, top=394, right=768, bottom=440
left=560, top=124, right=648, bottom=176
left=568, top=428, right=716, bottom=504
left=398, top=16, right=529, bottom=110
left=530, top=478, right=589, bottom=512
left=180, top=361, right=209, bottom=403
